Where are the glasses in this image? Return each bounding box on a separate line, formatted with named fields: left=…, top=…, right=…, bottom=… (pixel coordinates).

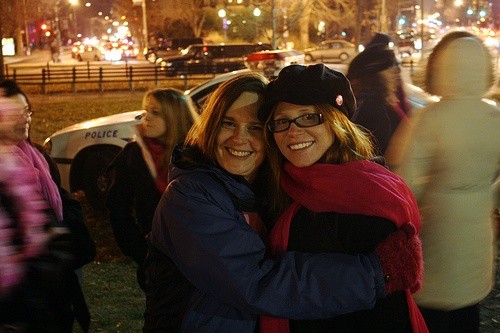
left=268, top=112, right=327, bottom=134
left=2, top=107, right=35, bottom=122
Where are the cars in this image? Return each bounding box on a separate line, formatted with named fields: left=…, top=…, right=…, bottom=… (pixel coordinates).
left=155, top=42, right=274, bottom=78
left=304, top=38, right=364, bottom=62
left=392, top=31, right=420, bottom=59
left=144, top=36, right=204, bottom=62
left=72, top=34, right=140, bottom=61
left=42, top=49, right=500, bottom=210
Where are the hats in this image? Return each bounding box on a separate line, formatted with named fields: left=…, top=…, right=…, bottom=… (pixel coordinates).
left=272, top=63, right=356, bottom=120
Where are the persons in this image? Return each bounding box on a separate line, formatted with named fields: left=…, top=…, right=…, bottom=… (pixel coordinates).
left=0, top=77, right=98, bottom=333
left=381, top=29, right=500, bottom=333
left=259, top=62, right=430, bottom=333
left=107, top=87, right=200, bottom=299
left=347, top=33, right=414, bottom=169
left=140, top=72, right=423, bottom=333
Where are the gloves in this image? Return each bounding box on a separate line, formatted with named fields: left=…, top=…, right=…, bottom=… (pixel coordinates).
left=373, top=221, right=423, bottom=293
